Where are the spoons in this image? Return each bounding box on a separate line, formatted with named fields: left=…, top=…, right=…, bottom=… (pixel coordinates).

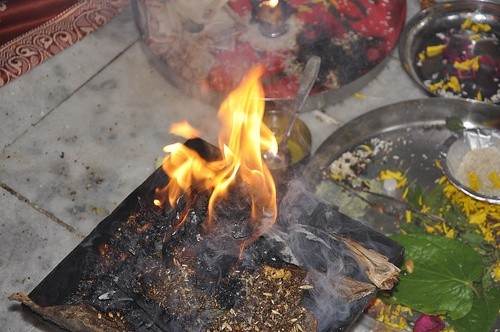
left=262, top=56, right=322, bottom=169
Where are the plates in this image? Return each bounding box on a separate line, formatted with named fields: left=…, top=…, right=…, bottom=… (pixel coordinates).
left=134, top=0, right=407, bottom=110
left=296, top=97, right=500, bottom=332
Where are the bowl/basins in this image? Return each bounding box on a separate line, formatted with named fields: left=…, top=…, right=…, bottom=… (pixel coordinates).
left=259, top=109, right=312, bottom=182
left=399, top=0, right=500, bottom=104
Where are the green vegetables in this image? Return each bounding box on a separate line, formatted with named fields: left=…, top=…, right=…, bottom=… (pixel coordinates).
left=377, top=182, right=499, bottom=331
